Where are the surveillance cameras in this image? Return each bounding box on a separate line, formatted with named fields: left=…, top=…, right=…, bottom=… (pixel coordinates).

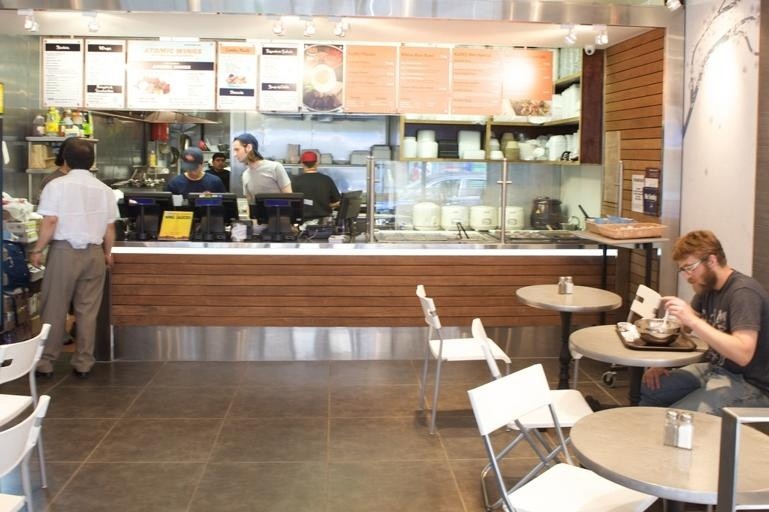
left=584, top=44, right=595, bottom=56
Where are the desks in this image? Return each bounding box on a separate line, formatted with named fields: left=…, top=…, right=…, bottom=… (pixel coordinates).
left=568, top=231, right=669, bottom=325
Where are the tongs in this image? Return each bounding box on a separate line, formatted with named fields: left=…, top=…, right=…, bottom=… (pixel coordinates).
left=457, top=223, right=470, bottom=239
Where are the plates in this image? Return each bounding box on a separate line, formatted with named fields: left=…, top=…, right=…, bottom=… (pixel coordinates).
left=371, top=144, right=391, bottom=160
left=350, top=150, right=370, bottom=166
left=320, top=153, right=330, bottom=165
left=303, top=45, right=343, bottom=113
left=457, top=130, right=485, bottom=160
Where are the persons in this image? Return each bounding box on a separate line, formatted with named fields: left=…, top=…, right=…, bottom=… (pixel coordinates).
left=29, top=137, right=120, bottom=379
left=291, top=151, right=341, bottom=235
left=203, top=152, right=231, bottom=192
left=160, top=146, right=226, bottom=236
left=638, top=229, right=769, bottom=417
left=232, top=133, right=293, bottom=226
left=40, top=139, right=73, bottom=199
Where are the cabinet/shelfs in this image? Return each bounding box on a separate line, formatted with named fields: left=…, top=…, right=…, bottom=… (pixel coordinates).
left=24, top=136, right=98, bottom=173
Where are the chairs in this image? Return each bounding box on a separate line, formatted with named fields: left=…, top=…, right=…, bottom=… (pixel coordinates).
left=415, top=284, right=511, bottom=437
left=572, top=284, right=661, bottom=388
left=466, top=363, right=667, bottom=511
left=471, top=318, right=594, bottom=511
left=0, top=395, right=51, bottom=511
left=0, top=323, right=52, bottom=489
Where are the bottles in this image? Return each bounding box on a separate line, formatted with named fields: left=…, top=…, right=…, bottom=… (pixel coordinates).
left=31, top=105, right=92, bottom=138
left=663, top=410, right=694, bottom=450
left=558, top=275, right=574, bottom=294
left=149, top=150, right=156, bottom=166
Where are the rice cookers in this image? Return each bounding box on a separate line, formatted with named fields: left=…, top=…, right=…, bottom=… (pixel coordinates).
left=441, top=204, right=469, bottom=230
left=470, top=206, right=497, bottom=232
left=499, top=206, right=525, bottom=230
left=412, top=202, right=441, bottom=230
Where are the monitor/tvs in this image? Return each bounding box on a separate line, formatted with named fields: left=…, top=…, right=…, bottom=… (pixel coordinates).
left=185, top=191, right=241, bottom=242
left=253, top=191, right=306, bottom=242
left=334, top=189, right=365, bottom=244
left=114, top=187, right=174, bottom=241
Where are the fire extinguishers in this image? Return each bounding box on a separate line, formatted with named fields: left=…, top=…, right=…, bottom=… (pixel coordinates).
left=152, top=122, right=169, bottom=141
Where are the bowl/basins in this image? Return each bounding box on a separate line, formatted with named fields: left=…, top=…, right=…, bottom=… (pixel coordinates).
left=636, top=317, right=679, bottom=344
left=416, top=129, right=438, bottom=159
left=288, top=143, right=301, bottom=164
left=490, top=131, right=520, bottom=160
left=334, top=158, right=348, bottom=166
left=549, top=130, right=580, bottom=160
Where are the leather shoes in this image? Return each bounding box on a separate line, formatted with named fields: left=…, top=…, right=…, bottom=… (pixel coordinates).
left=35, top=368, right=90, bottom=379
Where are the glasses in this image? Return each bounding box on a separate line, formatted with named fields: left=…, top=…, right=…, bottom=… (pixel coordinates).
left=677, top=254, right=710, bottom=273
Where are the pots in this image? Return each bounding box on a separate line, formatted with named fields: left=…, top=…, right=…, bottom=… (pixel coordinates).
left=531, top=196, right=563, bottom=230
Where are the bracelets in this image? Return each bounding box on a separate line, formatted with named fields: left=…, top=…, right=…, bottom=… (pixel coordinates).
left=27, top=248, right=44, bottom=255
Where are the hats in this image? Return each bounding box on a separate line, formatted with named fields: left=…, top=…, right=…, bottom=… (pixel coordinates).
left=238, top=133, right=264, bottom=160
left=182, top=147, right=202, bottom=170
left=301, top=152, right=317, bottom=162
